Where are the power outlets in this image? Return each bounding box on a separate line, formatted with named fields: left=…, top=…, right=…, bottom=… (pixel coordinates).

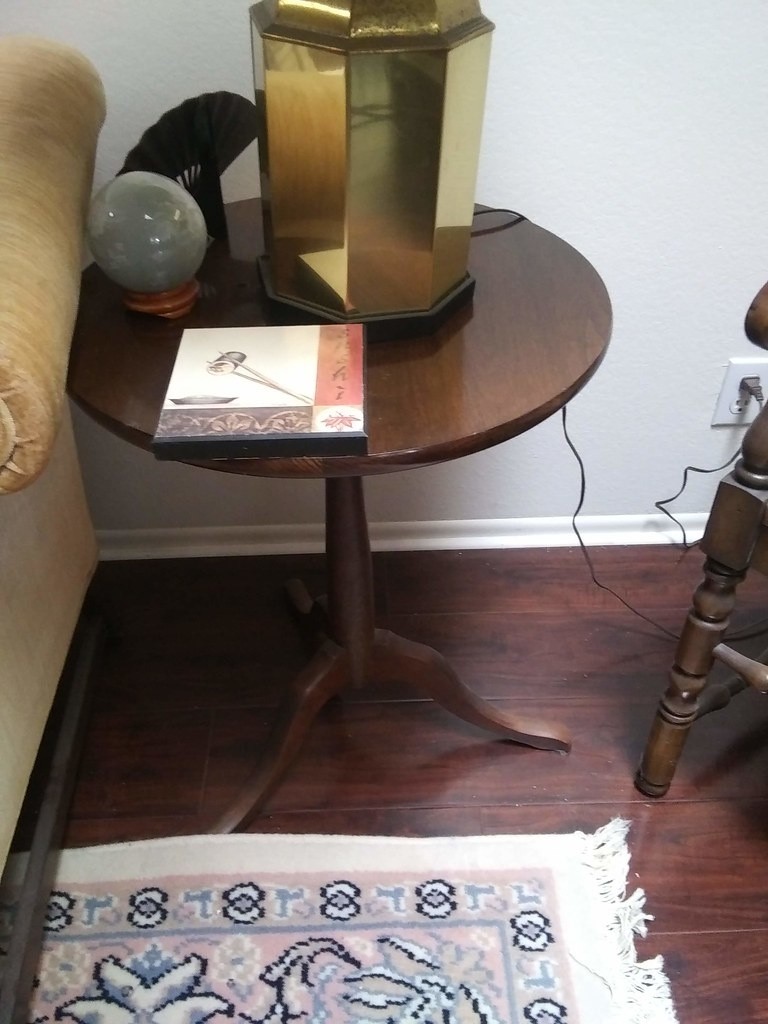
left=712, top=356, right=768, bottom=428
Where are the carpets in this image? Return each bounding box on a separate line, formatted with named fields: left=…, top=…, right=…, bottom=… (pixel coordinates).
left=0, top=810, right=680, bottom=1024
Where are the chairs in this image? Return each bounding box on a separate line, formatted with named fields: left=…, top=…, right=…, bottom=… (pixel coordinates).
left=631, top=281, right=768, bottom=797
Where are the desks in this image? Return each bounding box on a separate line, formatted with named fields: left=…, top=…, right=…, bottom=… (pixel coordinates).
left=65, top=182, right=614, bottom=830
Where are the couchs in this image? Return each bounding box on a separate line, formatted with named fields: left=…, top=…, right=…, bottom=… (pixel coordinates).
left=0, top=27, right=106, bottom=868
